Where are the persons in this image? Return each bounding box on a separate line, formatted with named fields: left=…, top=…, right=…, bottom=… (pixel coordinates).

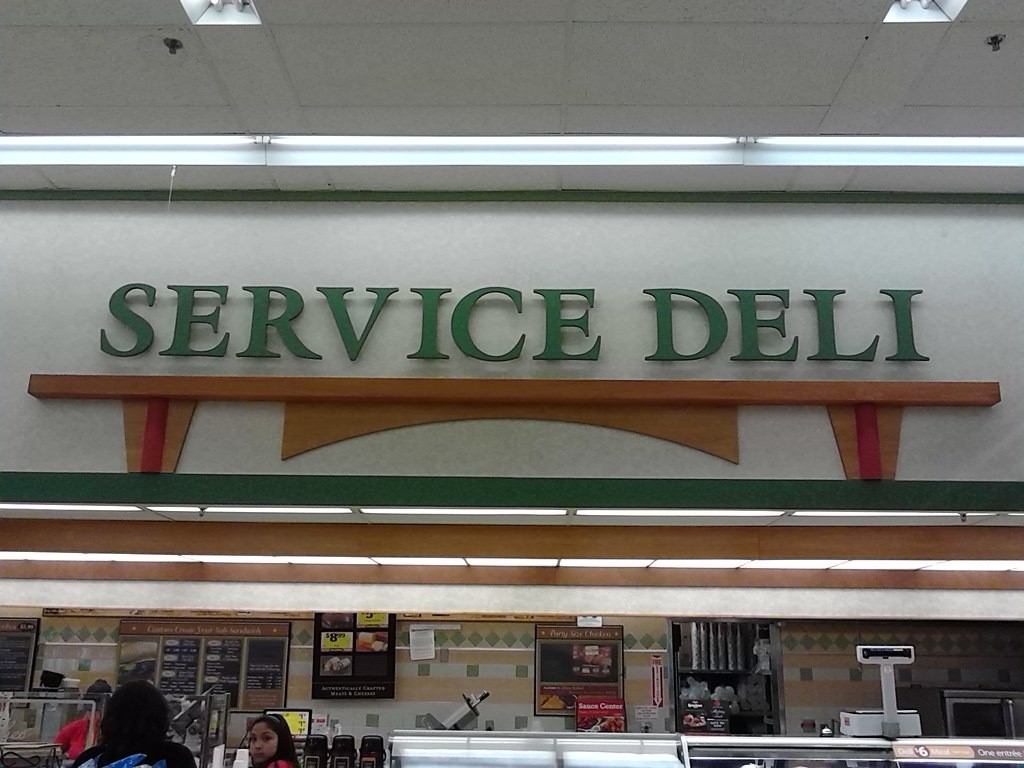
left=73, top=679, right=197, bottom=768
left=54, top=679, right=114, bottom=760
left=248, top=713, right=301, bottom=768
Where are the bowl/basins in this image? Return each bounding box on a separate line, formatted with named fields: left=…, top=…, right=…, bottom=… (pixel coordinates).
left=62, top=679, right=80, bottom=688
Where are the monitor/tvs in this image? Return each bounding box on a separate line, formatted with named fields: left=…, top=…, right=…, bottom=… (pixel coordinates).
left=264, top=708, right=312, bottom=742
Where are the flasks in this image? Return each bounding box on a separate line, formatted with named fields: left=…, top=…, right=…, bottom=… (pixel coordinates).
left=303, top=734, right=330, bottom=768
left=359, top=735, right=387, bottom=768
left=329, top=734, right=358, bottom=768
